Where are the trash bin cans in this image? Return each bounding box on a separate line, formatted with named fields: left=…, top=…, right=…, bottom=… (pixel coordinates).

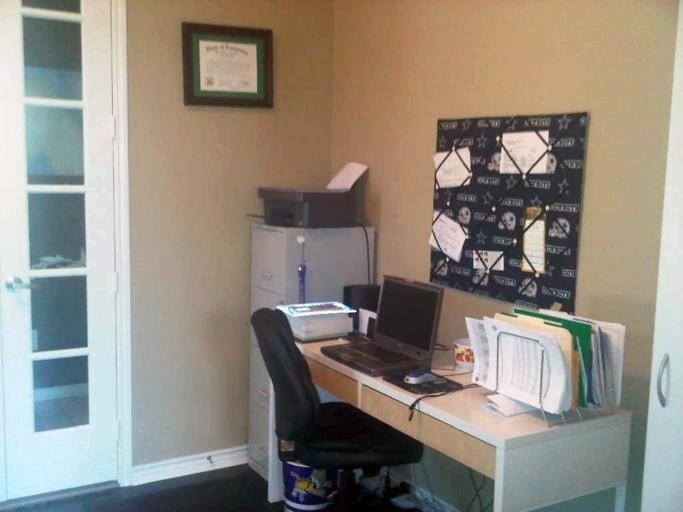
left=282, top=461, right=341, bottom=512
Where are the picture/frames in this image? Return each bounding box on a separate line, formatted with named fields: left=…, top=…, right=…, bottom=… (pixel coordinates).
left=181, top=23, right=274, bottom=111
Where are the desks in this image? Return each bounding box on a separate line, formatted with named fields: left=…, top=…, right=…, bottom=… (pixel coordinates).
left=267, top=337, right=632, bottom=512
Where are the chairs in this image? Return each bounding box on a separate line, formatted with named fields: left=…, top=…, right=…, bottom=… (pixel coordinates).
left=250, top=308, right=424, bottom=512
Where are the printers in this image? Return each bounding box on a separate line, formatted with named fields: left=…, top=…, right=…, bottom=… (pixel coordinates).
left=258, top=186, right=356, bottom=228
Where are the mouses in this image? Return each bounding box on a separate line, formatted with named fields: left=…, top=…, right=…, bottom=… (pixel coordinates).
left=403, top=368, right=437, bottom=386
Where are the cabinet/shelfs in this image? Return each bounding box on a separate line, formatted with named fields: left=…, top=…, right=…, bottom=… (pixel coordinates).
left=247, top=223, right=377, bottom=482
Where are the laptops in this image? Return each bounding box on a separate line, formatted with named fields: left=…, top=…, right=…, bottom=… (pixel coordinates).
left=320, top=275, right=444, bottom=377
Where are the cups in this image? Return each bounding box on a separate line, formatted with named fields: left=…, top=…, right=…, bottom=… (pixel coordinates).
left=453, top=337, right=473, bottom=371
